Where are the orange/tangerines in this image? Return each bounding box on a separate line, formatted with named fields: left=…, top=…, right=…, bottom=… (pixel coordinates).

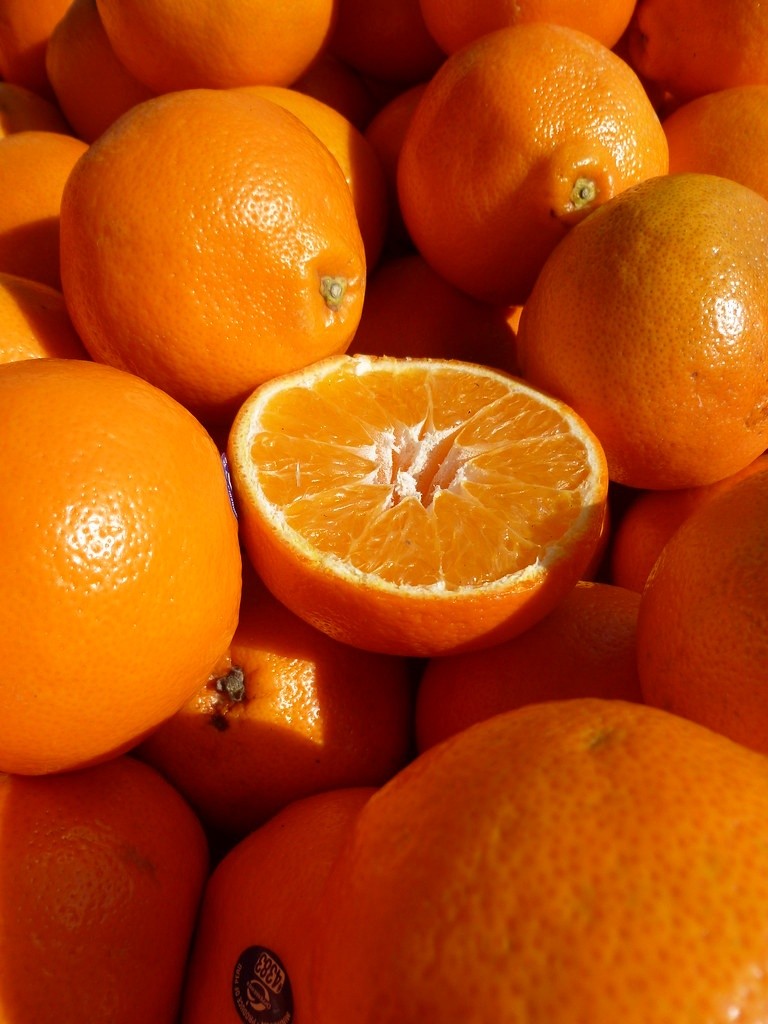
left=0, top=0, right=768, bottom=1024
